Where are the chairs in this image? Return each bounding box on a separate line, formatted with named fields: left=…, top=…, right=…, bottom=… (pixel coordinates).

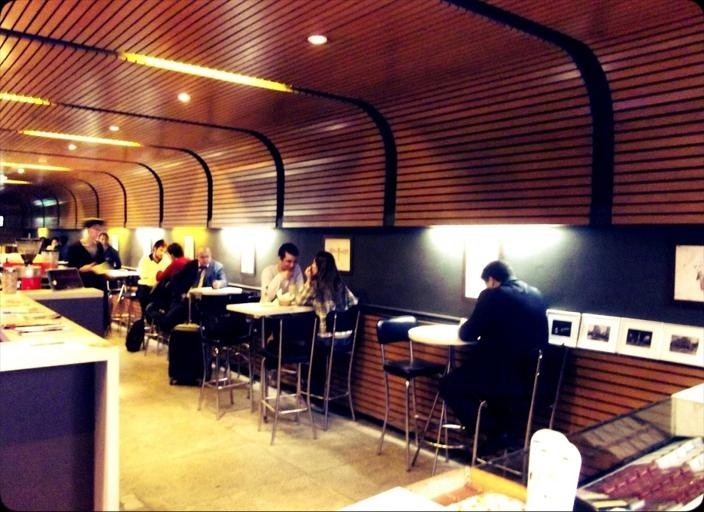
left=469, top=344, right=570, bottom=486
left=301, top=308, right=362, bottom=432
left=196, top=294, right=255, bottom=418
left=257, top=313, right=320, bottom=446
left=375, top=315, right=450, bottom=471
left=125, top=275, right=142, bottom=333
left=106, top=278, right=131, bottom=336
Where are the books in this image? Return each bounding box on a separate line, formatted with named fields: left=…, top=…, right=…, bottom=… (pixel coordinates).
left=90, top=264, right=138, bottom=280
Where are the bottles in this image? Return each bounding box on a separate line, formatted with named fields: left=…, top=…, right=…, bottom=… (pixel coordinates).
left=3, top=267, right=17, bottom=295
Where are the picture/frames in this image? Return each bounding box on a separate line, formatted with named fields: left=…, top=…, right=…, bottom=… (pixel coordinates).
left=667, top=239, right=704, bottom=308
left=320, top=234, right=355, bottom=276
left=461, top=244, right=502, bottom=304
left=239, top=250, right=257, bottom=277
left=576, top=312, right=621, bottom=354
left=616, top=317, right=665, bottom=361
left=545, top=308, right=581, bottom=349
left=659, top=323, right=704, bottom=368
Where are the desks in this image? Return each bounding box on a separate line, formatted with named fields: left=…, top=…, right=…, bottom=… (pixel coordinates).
left=137, top=278, right=159, bottom=288
left=190, top=286, right=244, bottom=386
left=407, top=324, right=477, bottom=466
left=225, top=300, right=315, bottom=422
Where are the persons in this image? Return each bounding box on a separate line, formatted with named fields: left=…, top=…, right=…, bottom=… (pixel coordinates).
left=67, top=219, right=110, bottom=330
left=99, top=231, right=121, bottom=270
left=438, top=259, right=548, bottom=434
left=155, top=242, right=192, bottom=287
left=297, top=250, right=357, bottom=349
left=181, top=246, right=227, bottom=300
left=260, top=243, right=304, bottom=303
left=135, top=240, right=172, bottom=312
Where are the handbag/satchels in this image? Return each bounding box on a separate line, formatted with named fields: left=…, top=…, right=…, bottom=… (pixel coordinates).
left=125, top=319, right=145, bottom=352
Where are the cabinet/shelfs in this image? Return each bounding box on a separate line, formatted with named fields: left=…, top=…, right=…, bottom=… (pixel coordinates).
left=0, top=284, right=121, bottom=512
left=335, top=381, right=704, bottom=512
